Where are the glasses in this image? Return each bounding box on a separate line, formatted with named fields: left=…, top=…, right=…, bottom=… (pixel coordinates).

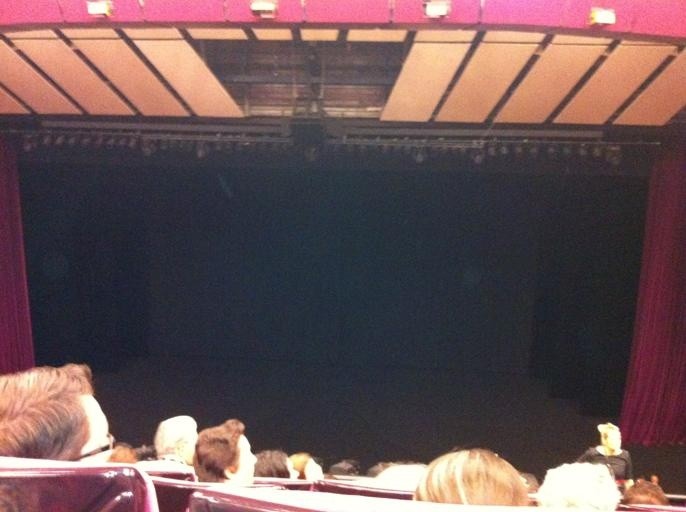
left=73, top=433, right=116, bottom=461
